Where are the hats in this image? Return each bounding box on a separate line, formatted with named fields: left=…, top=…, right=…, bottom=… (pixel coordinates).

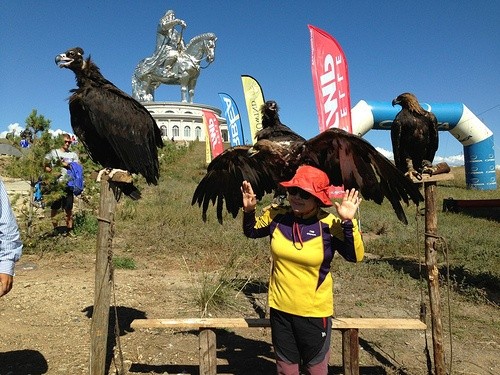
left=279, top=165, right=334, bottom=207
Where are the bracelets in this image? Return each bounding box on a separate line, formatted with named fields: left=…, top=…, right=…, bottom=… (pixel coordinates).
left=241, top=207, right=256, bottom=214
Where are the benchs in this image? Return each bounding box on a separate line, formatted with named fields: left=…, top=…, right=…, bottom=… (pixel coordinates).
left=129, top=317, right=426, bottom=375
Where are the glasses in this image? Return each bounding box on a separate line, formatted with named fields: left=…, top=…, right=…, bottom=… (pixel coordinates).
left=65, top=140, right=72, bottom=144
left=286, top=187, right=317, bottom=200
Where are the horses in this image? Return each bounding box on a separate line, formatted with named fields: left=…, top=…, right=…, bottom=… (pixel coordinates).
left=130, top=30, right=219, bottom=103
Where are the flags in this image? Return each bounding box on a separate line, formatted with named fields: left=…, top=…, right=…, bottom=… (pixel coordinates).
left=240, top=75, right=268, bottom=148
left=217, top=92, right=244, bottom=148
left=309, top=24, right=352, bottom=198
left=201, top=110, right=224, bottom=160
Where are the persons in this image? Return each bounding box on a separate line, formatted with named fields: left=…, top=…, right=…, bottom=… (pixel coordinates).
left=157, top=9, right=187, bottom=78
left=71, top=136, right=76, bottom=142
left=240, top=165, right=383, bottom=375
left=20, top=136, right=31, bottom=148
left=43, top=133, right=79, bottom=239
left=0, top=180, right=22, bottom=299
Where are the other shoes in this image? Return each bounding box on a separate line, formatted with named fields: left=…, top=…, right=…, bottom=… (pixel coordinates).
left=68, top=230, right=77, bottom=237
